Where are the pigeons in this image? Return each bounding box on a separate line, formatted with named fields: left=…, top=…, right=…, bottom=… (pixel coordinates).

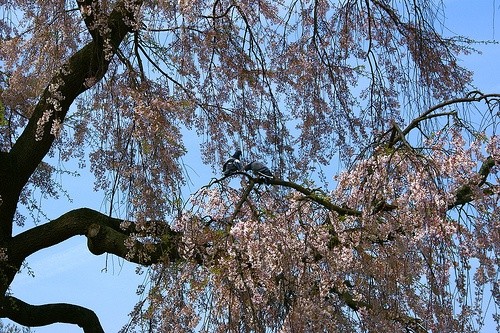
left=221, top=150, right=242, bottom=176
left=244, top=160, right=276, bottom=180
